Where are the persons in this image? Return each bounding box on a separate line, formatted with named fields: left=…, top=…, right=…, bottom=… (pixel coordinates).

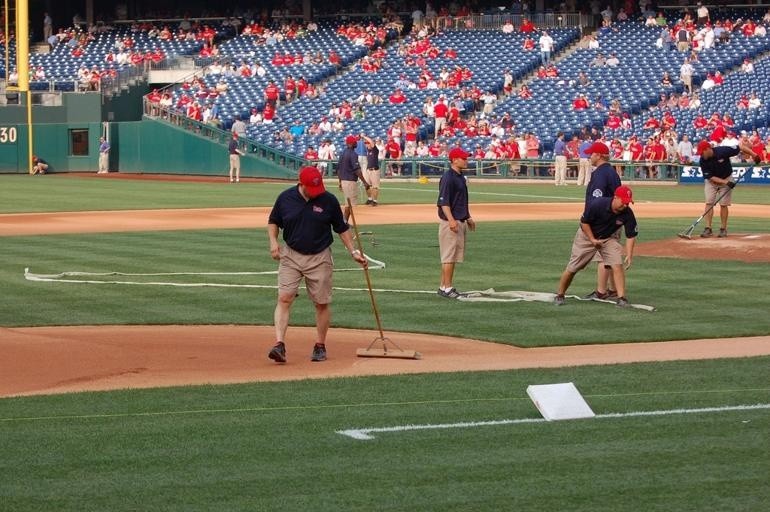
left=269, top=166, right=366, bottom=362
left=583, top=142, right=623, bottom=300
left=554, top=184, right=639, bottom=306
left=436, top=147, right=475, bottom=299
left=0, top=0, right=769, bottom=239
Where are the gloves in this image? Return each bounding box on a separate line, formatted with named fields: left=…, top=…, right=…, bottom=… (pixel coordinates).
left=726, top=181, right=735, bottom=189
left=755, top=155, right=761, bottom=164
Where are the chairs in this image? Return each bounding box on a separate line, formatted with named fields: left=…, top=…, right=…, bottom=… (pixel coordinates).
left=0, top=21, right=268, bottom=143
left=268, top=12, right=770, bottom=173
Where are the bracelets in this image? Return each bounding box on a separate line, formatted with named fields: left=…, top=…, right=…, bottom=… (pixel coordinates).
left=351, top=251, right=356, bottom=257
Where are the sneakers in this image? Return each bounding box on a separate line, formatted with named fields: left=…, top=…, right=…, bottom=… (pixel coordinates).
left=310, top=343, right=326, bottom=361
left=439, top=287, right=462, bottom=300
left=700, top=227, right=712, bottom=237
left=268, top=342, right=286, bottom=363
left=718, top=228, right=727, bottom=237
left=556, top=295, right=565, bottom=305
left=585, top=289, right=629, bottom=307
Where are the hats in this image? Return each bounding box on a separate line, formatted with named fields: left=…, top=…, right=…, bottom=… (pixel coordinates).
left=346, top=136, right=361, bottom=144
left=614, top=186, right=634, bottom=205
left=584, top=143, right=609, bottom=154
left=449, top=148, right=472, bottom=159
left=695, top=141, right=711, bottom=155
left=300, top=167, right=326, bottom=197
